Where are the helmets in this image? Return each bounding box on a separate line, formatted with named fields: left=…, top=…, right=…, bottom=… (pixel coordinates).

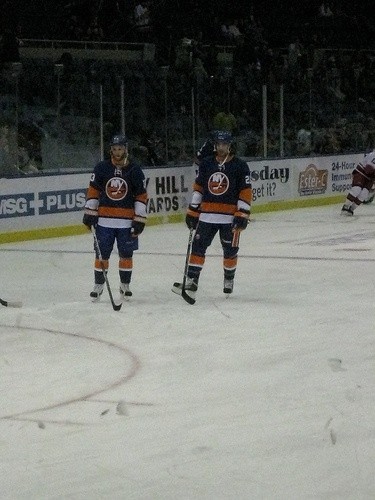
left=109, top=134, right=127, bottom=154
left=213, top=131, right=233, bottom=152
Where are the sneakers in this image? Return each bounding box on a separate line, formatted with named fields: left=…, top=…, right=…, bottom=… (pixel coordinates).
left=340, top=204, right=349, bottom=216
left=171, top=274, right=198, bottom=299
left=347, top=206, right=354, bottom=217
left=224, top=277, right=233, bottom=298
left=89, top=284, right=104, bottom=302
left=119, top=284, right=133, bottom=300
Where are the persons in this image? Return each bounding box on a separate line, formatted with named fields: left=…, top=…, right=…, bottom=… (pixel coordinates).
left=340, top=151, right=375, bottom=216
left=0, top=0, right=375, bottom=175
left=172, top=132, right=253, bottom=296
left=82, top=135, right=148, bottom=299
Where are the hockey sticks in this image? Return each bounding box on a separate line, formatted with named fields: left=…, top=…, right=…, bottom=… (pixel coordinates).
left=181, top=227, right=196, bottom=305
left=0, top=298, right=23, bottom=308
left=91, top=224, right=123, bottom=311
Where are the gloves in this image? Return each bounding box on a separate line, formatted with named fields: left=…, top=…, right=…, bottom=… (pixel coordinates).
left=82, top=208, right=99, bottom=230
left=186, top=205, right=201, bottom=230
left=130, top=216, right=146, bottom=238
left=231, top=209, right=251, bottom=230
